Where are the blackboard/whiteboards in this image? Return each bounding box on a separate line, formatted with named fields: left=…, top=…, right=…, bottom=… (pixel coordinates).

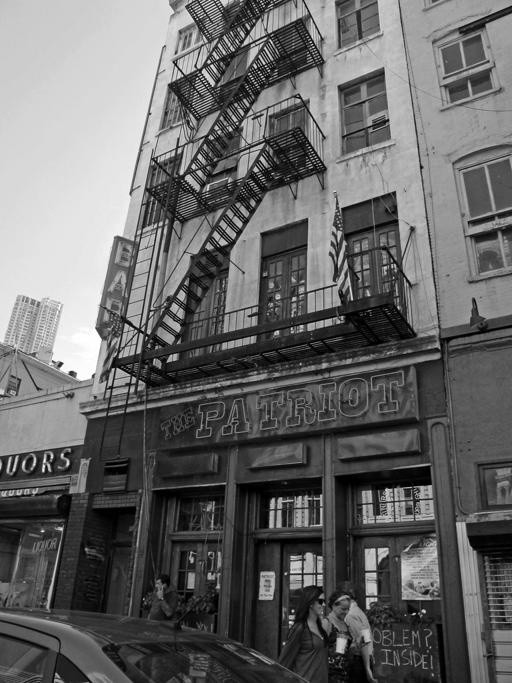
left=370, top=623, right=441, bottom=683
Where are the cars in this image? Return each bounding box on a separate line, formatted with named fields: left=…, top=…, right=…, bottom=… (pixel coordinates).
left=0, top=607, right=310, bottom=683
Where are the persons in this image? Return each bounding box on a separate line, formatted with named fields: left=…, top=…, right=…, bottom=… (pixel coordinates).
left=322, top=590, right=353, bottom=682
left=278, top=584, right=328, bottom=682
left=342, top=593, right=379, bottom=683
left=144, top=574, right=177, bottom=622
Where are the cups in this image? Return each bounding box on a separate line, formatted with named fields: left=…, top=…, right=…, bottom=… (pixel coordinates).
left=335, top=632, right=349, bottom=653
left=359, top=628, right=372, bottom=643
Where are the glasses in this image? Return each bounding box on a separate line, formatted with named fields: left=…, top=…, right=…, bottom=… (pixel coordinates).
left=316, top=598, right=326, bottom=604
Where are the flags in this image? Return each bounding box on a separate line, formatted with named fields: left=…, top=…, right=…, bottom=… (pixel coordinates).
left=326, top=199, right=357, bottom=305
